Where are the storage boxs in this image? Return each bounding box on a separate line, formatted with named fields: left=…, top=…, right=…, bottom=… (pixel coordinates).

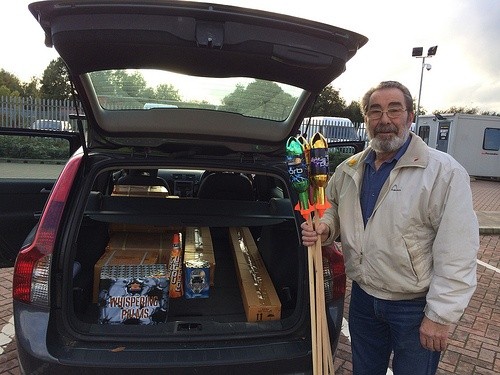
left=93, top=225, right=282, bottom=325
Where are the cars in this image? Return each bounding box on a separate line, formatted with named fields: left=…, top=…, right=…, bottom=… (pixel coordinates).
left=27, top=118, right=75, bottom=141
left=1, top=0, right=370, bottom=375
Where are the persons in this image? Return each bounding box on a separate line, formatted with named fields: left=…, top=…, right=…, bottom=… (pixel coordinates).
left=300, top=81, right=479, bottom=375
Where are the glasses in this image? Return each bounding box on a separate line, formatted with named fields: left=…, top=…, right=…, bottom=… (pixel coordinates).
left=363, top=108, right=411, bottom=119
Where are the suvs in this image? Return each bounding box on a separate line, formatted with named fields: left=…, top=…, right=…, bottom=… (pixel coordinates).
left=298, top=115, right=360, bottom=158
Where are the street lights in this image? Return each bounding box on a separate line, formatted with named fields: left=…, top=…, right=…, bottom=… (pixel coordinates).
left=412, top=45, right=438, bottom=135
